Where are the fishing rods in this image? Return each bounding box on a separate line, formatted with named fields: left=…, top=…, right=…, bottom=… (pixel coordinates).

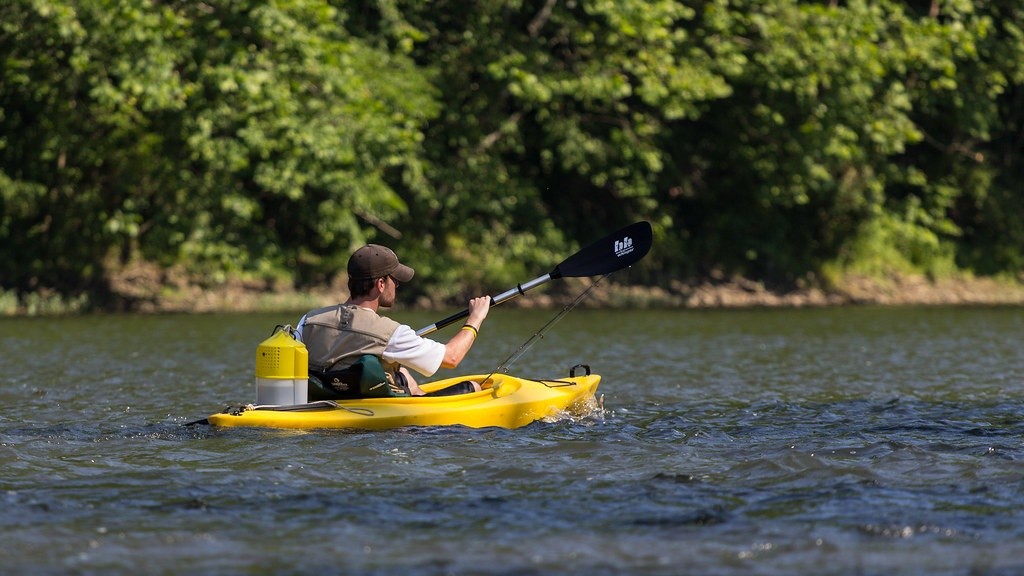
left=479, top=274, right=612, bottom=387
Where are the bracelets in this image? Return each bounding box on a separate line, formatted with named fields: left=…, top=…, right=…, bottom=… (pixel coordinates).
left=462, top=325, right=477, bottom=337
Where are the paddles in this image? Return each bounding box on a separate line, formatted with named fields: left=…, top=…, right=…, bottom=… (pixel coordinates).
left=180, top=220, right=653, bottom=427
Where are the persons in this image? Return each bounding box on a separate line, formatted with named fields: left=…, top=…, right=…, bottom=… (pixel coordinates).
left=293, top=244, right=491, bottom=398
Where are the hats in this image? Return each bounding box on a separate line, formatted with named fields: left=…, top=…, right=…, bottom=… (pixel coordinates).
left=347, top=244, right=415, bottom=283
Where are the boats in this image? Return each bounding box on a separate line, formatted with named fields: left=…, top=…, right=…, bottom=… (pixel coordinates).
left=206, top=365, right=601, bottom=434
